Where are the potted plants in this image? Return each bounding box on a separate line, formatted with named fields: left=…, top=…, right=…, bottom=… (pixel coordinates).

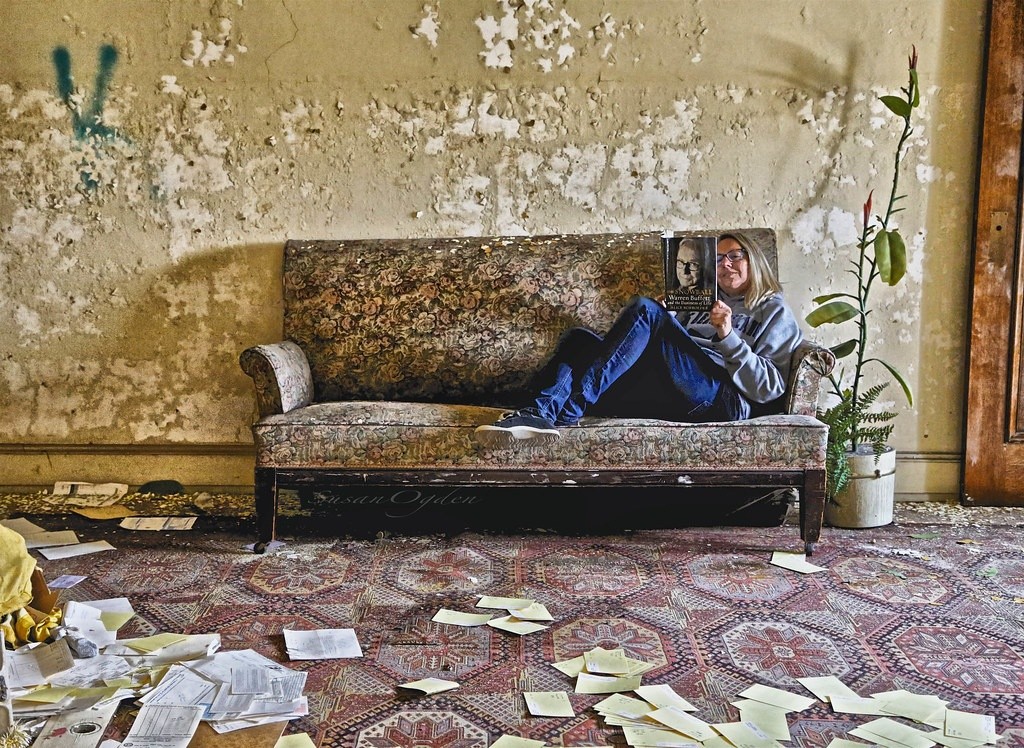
left=807, top=45, right=923, bottom=533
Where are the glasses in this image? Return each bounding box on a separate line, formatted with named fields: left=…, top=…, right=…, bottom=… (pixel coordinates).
left=675, top=260, right=699, bottom=271
left=717, top=249, right=746, bottom=266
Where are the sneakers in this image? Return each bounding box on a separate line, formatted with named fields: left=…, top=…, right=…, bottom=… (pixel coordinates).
left=474, top=411, right=560, bottom=449
left=498, top=409, right=580, bottom=427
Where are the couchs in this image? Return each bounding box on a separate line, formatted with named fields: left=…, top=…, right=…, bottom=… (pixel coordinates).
left=240, top=229, right=837, bottom=562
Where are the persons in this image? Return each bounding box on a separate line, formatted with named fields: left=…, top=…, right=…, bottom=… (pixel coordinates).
left=474, top=231, right=803, bottom=449
left=667, top=238, right=715, bottom=294
